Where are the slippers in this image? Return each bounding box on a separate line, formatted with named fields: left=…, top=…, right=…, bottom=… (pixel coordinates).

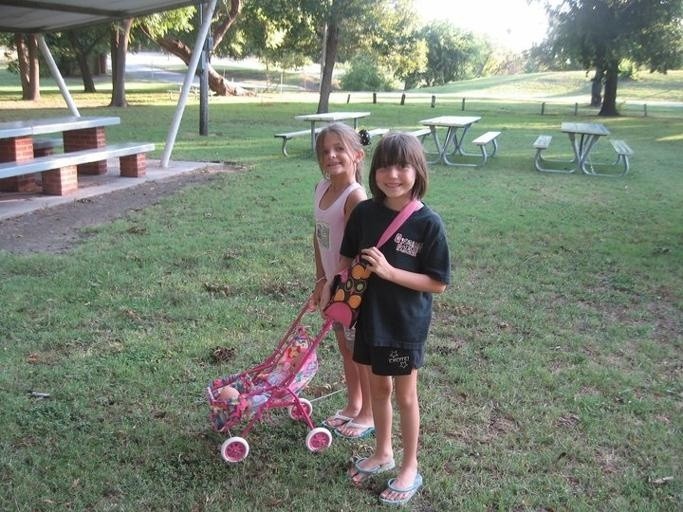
left=333, top=418, right=374, bottom=439
left=346, top=457, right=395, bottom=485
left=378, top=473, right=422, bottom=506
left=321, top=409, right=352, bottom=428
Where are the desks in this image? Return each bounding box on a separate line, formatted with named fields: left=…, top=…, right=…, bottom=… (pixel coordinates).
left=419, top=116, right=481, bottom=166
left=560, top=122, right=610, bottom=176
left=293, top=111, right=371, bottom=137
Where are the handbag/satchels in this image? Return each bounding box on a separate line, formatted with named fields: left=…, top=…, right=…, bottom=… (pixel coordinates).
left=322, top=197, right=422, bottom=328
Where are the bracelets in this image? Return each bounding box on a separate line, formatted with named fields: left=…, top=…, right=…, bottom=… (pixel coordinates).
left=314, top=275, right=325, bottom=284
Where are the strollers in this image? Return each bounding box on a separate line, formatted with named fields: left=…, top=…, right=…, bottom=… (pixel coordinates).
left=206, top=301, right=334, bottom=462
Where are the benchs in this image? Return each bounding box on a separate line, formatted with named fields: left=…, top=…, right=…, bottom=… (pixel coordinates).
left=580, top=139, right=633, bottom=178
left=442, top=131, right=501, bottom=167
left=274, top=126, right=326, bottom=157
left=408, top=129, right=458, bottom=165
left=366, top=128, right=389, bottom=139
left=531, top=134, right=582, bottom=174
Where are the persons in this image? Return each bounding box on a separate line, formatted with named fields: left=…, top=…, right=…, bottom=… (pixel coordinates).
left=334, top=134, right=449, bottom=505
left=310, top=121, right=374, bottom=437
